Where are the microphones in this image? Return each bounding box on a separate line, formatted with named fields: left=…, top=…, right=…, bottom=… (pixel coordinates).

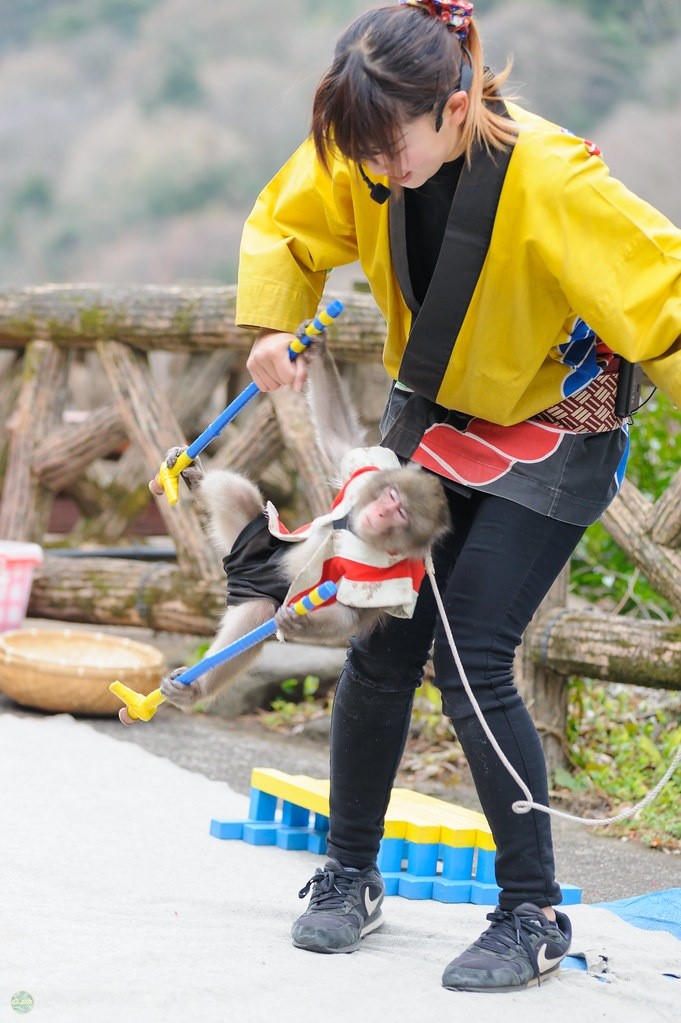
left=356, top=162, right=391, bottom=205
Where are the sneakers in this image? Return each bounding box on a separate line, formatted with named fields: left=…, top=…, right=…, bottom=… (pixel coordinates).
left=441, top=900, right=573, bottom=994
left=291, top=856, right=388, bottom=957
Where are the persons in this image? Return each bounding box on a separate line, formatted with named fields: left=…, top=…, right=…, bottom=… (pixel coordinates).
left=232, top=1, right=679, bottom=993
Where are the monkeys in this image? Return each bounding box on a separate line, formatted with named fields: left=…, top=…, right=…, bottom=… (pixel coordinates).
left=158, top=318, right=454, bottom=708
